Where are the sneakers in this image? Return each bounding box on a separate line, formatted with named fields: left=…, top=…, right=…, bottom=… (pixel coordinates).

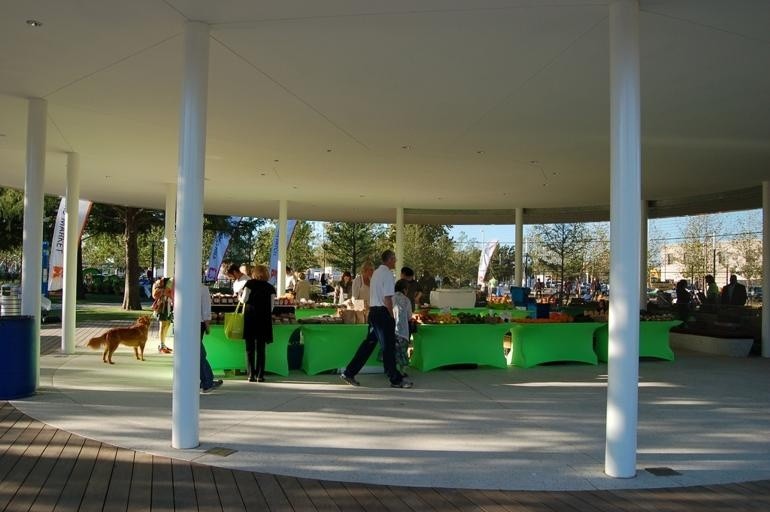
left=200, top=379, right=223, bottom=393
left=391, top=380, right=413, bottom=389
left=157, top=345, right=173, bottom=355
left=340, top=373, right=360, bottom=386
left=248, top=375, right=265, bottom=382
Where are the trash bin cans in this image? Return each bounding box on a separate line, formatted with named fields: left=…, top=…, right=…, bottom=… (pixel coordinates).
left=0, top=284, right=22, bottom=316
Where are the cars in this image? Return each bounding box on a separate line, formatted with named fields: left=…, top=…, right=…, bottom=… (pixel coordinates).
left=546, top=279, right=703, bottom=307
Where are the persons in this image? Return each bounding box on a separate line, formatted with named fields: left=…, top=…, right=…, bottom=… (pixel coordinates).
left=545, top=279, right=552, bottom=289
left=236, top=265, right=277, bottom=382
left=704, top=273, right=719, bottom=304
left=440, top=277, right=454, bottom=289
left=394, top=266, right=423, bottom=314
left=417, top=270, right=438, bottom=305
left=461, top=279, right=473, bottom=289
left=533, top=278, right=545, bottom=298
left=721, top=274, right=748, bottom=305
left=601, top=282, right=607, bottom=299
left=563, top=279, right=602, bottom=306
left=320, top=273, right=329, bottom=294
left=488, top=274, right=499, bottom=294
left=223, top=261, right=312, bottom=303
left=675, top=279, right=694, bottom=304
left=333, top=271, right=352, bottom=307
left=200, top=270, right=224, bottom=392
left=352, top=261, right=376, bottom=305
left=340, top=250, right=415, bottom=389
left=656, top=290, right=672, bottom=306
left=378, top=278, right=415, bottom=380
left=151, top=275, right=173, bottom=353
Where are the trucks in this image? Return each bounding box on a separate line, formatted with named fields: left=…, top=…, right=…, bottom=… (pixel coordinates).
left=302, top=266, right=345, bottom=282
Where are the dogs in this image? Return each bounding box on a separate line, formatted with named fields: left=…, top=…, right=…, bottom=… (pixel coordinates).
left=85, top=314, right=152, bottom=364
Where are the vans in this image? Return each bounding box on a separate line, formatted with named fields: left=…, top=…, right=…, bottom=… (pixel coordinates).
left=745, top=284, right=763, bottom=301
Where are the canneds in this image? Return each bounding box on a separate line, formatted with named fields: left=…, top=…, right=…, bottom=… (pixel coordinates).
left=213, top=294, right=238, bottom=304
left=274, top=298, right=288, bottom=305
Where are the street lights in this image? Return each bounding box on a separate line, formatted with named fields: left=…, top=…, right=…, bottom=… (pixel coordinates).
left=523, top=252, right=530, bottom=288
left=149, top=237, right=159, bottom=283
left=480, top=229, right=485, bottom=247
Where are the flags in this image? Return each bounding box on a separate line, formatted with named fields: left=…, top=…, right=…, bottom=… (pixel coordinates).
left=477, top=239, right=498, bottom=285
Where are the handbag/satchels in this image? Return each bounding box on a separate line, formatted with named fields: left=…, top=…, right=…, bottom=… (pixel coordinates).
left=224, top=300, right=246, bottom=339
left=152, top=300, right=166, bottom=313
left=409, top=320, right=417, bottom=333
